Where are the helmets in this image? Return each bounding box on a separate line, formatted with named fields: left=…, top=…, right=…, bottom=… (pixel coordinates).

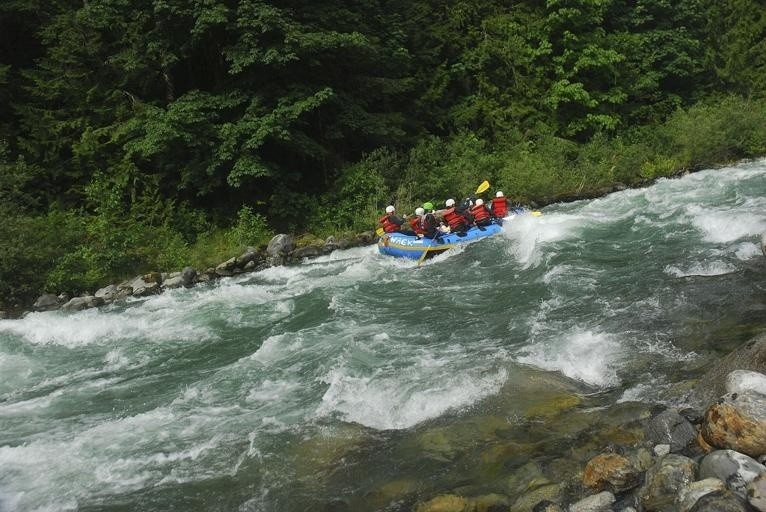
left=386, top=205, right=395, bottom=213
left=445, top=198, right=455, bottom=207
left=423, top=203, right=433, bottom=209
left=475, top=198, right=483, bottom=206
left=415, top=207, right=424, bottom=215
left=495, top=191, right=504, bottom=198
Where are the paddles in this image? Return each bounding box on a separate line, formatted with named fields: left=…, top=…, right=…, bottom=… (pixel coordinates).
left=417, top=225, right=441, bottom=265
left=461, top=180, right=489, bottom=208
left=375, top=227, right=384, bottom=236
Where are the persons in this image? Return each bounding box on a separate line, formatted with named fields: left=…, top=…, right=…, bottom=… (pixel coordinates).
left=467, top=197, right=491, bottom=227
left=441, top=198, right=471, bottom=233
left=490, top=190, right=513, bottom=221
left=419, top=201, right=447, bottom=240
left=380, top=204, right=417, bottom=236
left=462, top=201, right=475, bottom=225
left=408, top=206, right=426, bottom=237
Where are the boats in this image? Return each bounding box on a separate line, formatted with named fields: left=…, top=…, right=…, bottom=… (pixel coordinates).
left=374, top=206, right=528, bottom=263
